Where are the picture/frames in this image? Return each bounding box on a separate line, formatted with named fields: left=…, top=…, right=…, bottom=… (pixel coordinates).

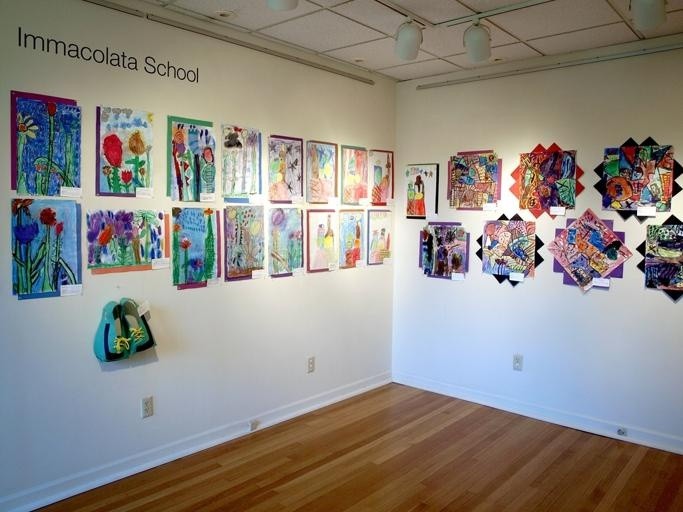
left=509, top=143, right=586, bottom=219
left=636, top=215, right=683, bottom=302
left=475, top=213, right=544, bottom=287
left=419, top=222, right=470, bottom=280
left=167, top=115, right=215, bottom=201
left=267, top=135, right=302, bottom=205
left=341, top=145, right=368, bottom=205
left=10, top=199, right=83, bottom=301
left=94, top=107, right=152, bottom=198
left=268, top=207, right=305, bottom=278
left=447, top=150, right=502, bottom=211
left=367, top=210, right=391, bottom=265
left=305, top=140, right=338, bottom=204
left=592, top=136, right=682, bottom=224
left=546, top=209, right=634, bottom=293
left=306, top=209, right=336, bottom=273
left=173, top=207, right=222, bottom=290
left=224, top=206, right=265, bottom=282
left=85, top=207, right=172, bottom=276
left=10, top=89, right=81, bottom=196
left=369, top=149, right=394, bottom=205
left=340, top=210, right=365, bottom=269
left=220, top=122, right=262, bottom=200
left=405, top=163, right=439, bottom=219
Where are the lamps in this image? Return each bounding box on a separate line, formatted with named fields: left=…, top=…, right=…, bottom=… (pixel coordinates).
left=395, top=17, right=425, bottom=66
left=463, top=19, right=494, bottom=62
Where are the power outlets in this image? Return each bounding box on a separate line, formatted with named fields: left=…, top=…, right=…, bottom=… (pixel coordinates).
left=513, top=354, right=524, bottom=371
left=307, top=355, right=315, bottom=374
left=141, top=396, right=154, bottom=418
